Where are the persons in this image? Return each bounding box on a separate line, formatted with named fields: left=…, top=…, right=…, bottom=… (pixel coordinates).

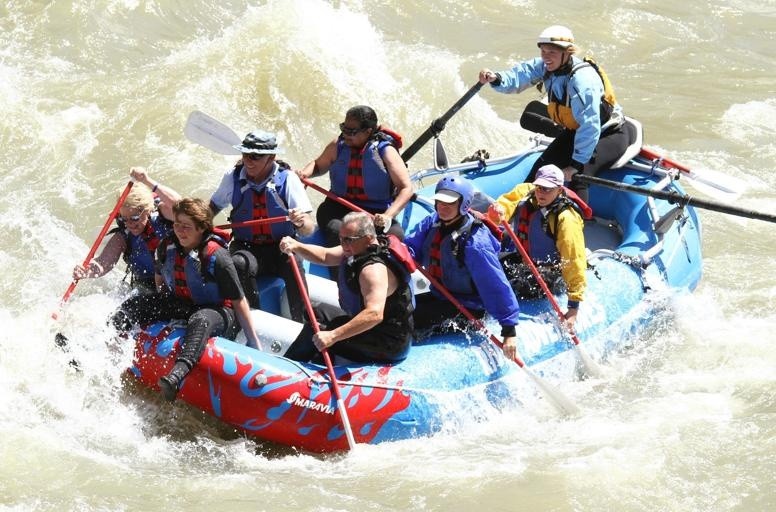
left=73, top=165, right=182, bottom=292
left=54, top=198, right=262, bottom=403
left=403, top=175, right=519, bottom=361
left=279, top=213, right=415, bottom=367
left=207, top=130, right=309, bottom=323
left=486, top=162, right=592, bottom=330
left=295, top=106, right=414, bottom=282
left=479, top=25, right=629, bottom=206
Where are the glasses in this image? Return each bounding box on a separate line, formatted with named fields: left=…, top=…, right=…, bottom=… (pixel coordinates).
left=242, top=152, right=267, bottom=162
left=340, top=124, right=367, bottom=136
left=537, top=187, right=557, bottom=193
left=117, top=209, right=145, bottom=222
left=336, top=234, right=367, bottom=244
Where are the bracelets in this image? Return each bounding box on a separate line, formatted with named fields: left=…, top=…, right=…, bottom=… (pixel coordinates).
left=152, top=185, right=158, bottom=193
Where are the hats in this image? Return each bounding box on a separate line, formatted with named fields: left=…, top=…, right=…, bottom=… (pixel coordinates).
left=532, top=163, right=564, bottom=187
left=233, top=132, right=287, bottom=154
left=435, top=191, right=461, bottom=204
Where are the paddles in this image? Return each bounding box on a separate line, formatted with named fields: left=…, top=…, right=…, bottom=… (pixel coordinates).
left=185, top=111, right=375, bottom=220
left=640, top=146, right=748, bottom=204
left=414, top=261, right=581, bottom=419
left=502, top=220, right=609, bottom=379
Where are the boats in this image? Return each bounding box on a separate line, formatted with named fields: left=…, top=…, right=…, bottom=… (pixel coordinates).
left=122, top=144, right=707, bottom=455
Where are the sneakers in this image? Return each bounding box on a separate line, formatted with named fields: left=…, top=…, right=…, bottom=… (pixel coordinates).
left=159, top=374, right=178, bottom=406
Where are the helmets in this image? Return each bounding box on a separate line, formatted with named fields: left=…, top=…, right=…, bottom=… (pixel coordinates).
left=435, top=177, right=474, bottom=215
left=537, top=26, right=574, bottom=49
